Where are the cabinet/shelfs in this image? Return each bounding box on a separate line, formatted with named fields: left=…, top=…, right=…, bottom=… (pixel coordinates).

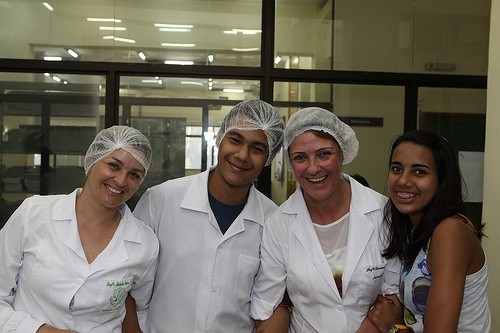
left=0, top=81, right=100, bottom=228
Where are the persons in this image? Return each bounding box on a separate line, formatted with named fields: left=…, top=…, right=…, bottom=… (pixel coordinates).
left=121, top=99, right=292, bottom=333
left=250, top=107, right=405, bottom=333
left=0, top=125, right=159, bottom=333
left=365, top=129, right=492, bottom=333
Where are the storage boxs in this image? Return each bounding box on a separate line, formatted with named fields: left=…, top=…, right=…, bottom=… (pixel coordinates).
left=0, top=163, right=87, bottom=193
left=5, top=123, right=97, bottom=151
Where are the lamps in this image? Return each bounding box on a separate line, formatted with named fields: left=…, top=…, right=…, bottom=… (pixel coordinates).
left=65, top=48, right=80, bottom=59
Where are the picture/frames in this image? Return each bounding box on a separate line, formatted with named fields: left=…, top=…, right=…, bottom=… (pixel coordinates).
left=0, top=81, right=98, bottom=117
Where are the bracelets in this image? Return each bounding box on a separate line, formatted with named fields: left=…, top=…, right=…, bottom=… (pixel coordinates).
left=388, top=325, right=407, bottom=333
left=278, top=304, right=292, bottom=314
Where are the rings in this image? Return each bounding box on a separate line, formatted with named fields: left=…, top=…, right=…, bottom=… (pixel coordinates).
left=369, top=306, right=375, bottom=312
